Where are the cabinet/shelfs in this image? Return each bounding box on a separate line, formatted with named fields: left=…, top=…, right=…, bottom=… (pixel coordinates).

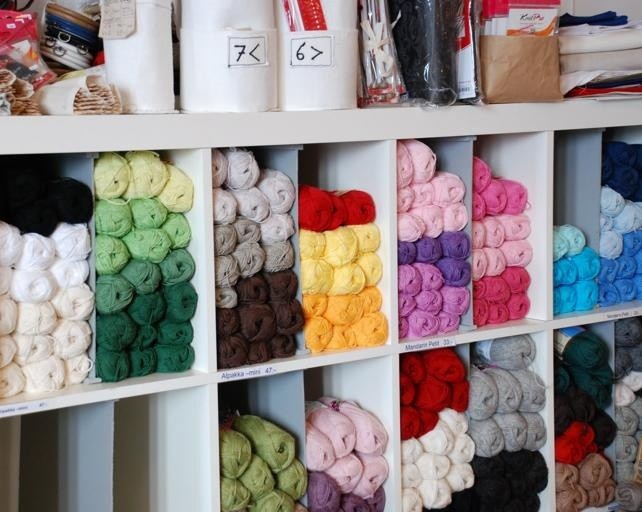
left=0, top=94, right=642, bottom=512
